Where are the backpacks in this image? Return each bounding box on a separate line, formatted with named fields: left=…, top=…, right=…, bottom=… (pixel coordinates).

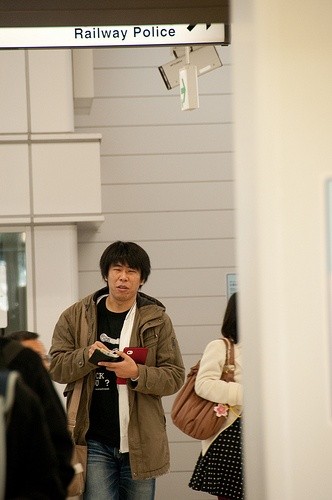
left=0, top=339, right=51, bottom=500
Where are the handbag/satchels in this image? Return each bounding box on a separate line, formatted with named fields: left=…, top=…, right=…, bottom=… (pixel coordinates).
left=172, top=337, right=236, bottom=440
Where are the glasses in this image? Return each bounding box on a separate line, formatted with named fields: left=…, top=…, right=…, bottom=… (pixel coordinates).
left=41, top=355, right=53, bottom=365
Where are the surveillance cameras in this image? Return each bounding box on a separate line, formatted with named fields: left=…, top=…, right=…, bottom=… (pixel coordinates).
left=158, top=45, right=222, bottom=90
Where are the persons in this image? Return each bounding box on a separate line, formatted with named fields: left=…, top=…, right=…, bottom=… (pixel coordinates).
left=0, top=331, right=75, bottom=500
left=50, top=241, right=185, bottom=500
left=188, top=292, right=244, bottom=500
